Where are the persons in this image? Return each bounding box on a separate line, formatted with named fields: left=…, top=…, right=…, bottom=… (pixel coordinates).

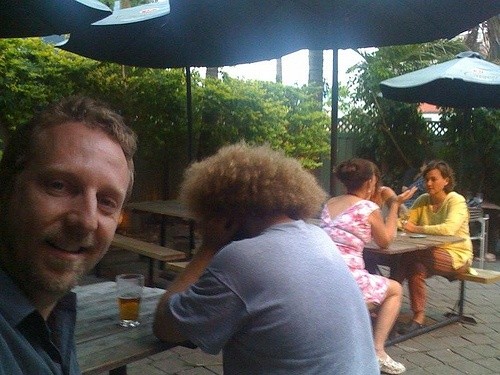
left=320, top=158, right=419, bottom=375
left=363, top=163, right=407, bottom=276
left=153, top=137, right=380, bottom=375
left=0, top=98, right=135, bottom=375
left=378, top=162, right=474, bottom=335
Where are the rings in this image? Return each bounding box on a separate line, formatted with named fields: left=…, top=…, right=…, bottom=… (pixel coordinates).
left=402, top=220, right=408, bottom=224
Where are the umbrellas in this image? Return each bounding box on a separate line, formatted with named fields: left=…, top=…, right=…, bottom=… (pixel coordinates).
left=0, top=0, right=499, bottom=196
left=379, top=51, right=500, bottom=194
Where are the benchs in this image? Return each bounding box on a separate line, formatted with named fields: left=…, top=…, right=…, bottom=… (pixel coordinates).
left=445, top=266, right=500, bottom=325
left=95, top=233, right=185, bottom=288
left=163, top=262, right=377, bottom=313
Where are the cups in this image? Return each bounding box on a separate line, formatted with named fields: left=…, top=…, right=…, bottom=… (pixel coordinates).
left=116, top=274, right=145, bottom=327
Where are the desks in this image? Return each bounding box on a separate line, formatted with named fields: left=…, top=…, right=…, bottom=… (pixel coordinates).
left=60, top=281, right=183, bottom=375
left=128, top=197, right=205, bottom=258
left=305, top=214, right=466, bottom=347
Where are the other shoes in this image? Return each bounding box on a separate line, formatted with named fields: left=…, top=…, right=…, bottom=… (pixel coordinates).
left=376, top=354, right=406, bottom=374
left=399, top=319, right=425, bottom=336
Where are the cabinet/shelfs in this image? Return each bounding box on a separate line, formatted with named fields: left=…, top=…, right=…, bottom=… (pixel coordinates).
left=465, top=205, right=491, bottom=270
